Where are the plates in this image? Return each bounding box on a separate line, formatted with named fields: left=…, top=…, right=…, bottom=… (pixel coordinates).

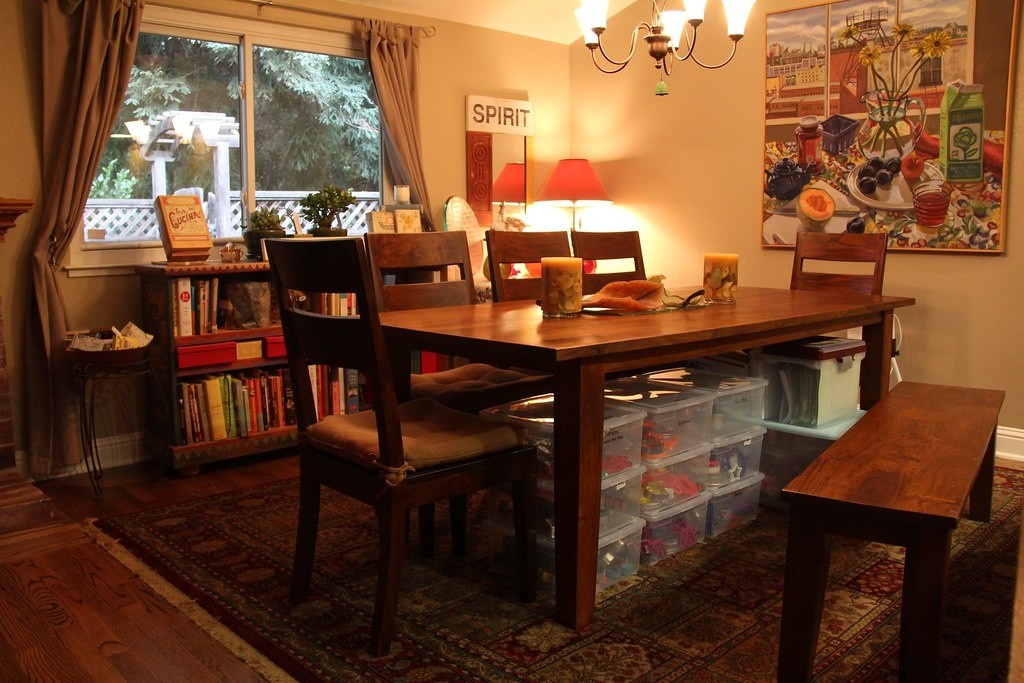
left=847, top=161, right=946, bottom=209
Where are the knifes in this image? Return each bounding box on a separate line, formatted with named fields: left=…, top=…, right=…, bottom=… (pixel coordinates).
left=763, top=208, right=860, bottom=218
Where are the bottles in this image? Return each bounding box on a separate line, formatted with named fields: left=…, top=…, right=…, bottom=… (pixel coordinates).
left=795, top=115, right=825, bottom=177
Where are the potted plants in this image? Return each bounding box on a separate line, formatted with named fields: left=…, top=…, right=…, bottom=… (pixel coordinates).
left=300, top=184, right=357, bottom=237
left=240, top=208, right=286, bottom=260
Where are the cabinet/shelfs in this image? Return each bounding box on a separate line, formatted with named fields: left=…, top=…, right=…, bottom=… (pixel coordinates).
left=134, top=259, right=449, bottom=477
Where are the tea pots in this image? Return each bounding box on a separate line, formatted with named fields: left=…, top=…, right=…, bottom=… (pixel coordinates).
left=765, top=158, right=817, bottom=201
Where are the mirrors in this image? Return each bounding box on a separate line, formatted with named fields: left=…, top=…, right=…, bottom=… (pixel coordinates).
left=492, top=133, right=526, bottom=229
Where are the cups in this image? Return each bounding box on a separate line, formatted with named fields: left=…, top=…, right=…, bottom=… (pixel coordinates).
left=703, top=253, right=740, bottom=306
left=219, top=248, right=244, bottom=262
left=911, top=179, right=952, bottom=228
left=540, top=255, right=582, bottom=318
left=393, top=183, right=410, bottom=204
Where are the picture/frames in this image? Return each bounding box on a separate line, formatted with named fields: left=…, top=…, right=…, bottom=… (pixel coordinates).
left=759, top=0, right=1022, bottom=253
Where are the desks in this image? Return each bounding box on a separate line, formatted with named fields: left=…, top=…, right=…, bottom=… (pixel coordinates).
left=344, top=285, right=916, bottom=632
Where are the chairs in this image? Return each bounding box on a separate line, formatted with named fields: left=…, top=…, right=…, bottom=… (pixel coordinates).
left=789, top=231, right=888, bottom=294
left=487, top=230, right=571, bottom=301
left=263, top=238, right=540, bottom=650
left=364, top=231, right=541, bottom=415
left=570, top=228, right=647, bottom=297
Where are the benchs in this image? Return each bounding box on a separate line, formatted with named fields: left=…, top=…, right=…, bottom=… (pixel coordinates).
left=777, top=382, right=1006, bottom=683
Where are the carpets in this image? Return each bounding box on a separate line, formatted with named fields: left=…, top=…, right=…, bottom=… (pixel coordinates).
left=79, top=466, right=1024, bottom=683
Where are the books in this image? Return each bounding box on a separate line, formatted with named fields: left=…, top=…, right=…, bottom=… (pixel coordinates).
left=177, top=352, right=470, bottom=445
left=168, top=279, right=356, bottom=337
left=383, top=264, right=462, bottom=286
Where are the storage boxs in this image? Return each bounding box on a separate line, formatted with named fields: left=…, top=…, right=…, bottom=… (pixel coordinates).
left=476, top=336, right=871, bottom=595
left=177, top=342, right=237, bottom=370
left=263, top=336, right=288, bottom=359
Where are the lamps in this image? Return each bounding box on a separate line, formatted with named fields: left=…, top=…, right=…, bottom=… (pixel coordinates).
left=533, top=158, right=615, bottom=275
left=576, top=0, right=757, bottom=96
left=492, top=162, right=527, bottom=231
